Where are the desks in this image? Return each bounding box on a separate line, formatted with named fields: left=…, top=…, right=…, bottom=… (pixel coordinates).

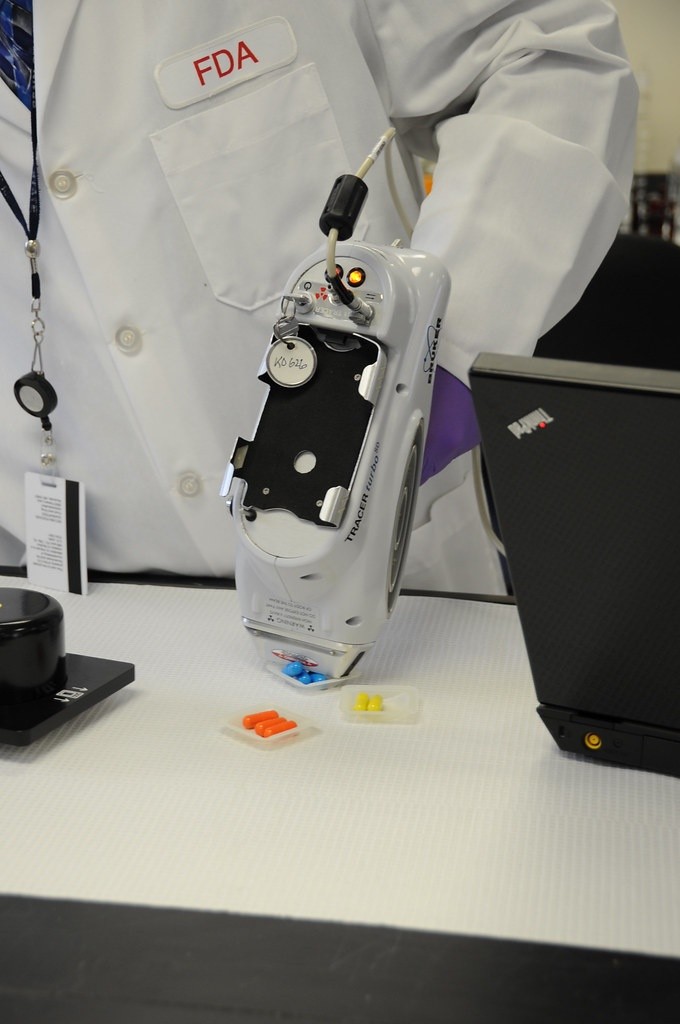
left=1, top=565, right=680, bottom=1024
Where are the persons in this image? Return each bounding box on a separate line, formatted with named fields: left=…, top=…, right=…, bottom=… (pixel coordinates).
left=0, top=0, right=641, bottom=577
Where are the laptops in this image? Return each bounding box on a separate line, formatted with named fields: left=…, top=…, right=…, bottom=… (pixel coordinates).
left=468, top=349, right=680, bottom=781
